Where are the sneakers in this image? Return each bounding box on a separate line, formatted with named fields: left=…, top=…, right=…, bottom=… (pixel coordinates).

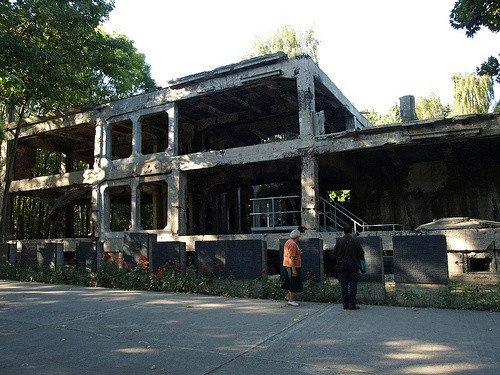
left=285, top=297, right=290, bottom=302
left=288, top=301, right=300, bottom=306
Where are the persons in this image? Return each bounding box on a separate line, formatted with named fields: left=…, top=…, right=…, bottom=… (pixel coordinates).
left=283, top=230, right=303, bottom=307
left=334, top=226, right=366, bottom=310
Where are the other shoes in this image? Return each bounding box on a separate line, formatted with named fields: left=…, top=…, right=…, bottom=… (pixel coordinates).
left=343, top=304, right=360, bottom=310
